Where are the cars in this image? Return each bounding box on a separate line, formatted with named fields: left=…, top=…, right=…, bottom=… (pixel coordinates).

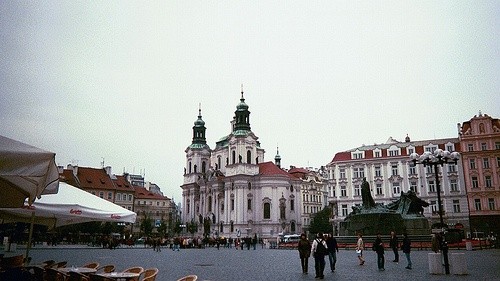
left=138, top=237, right=147, bottom=244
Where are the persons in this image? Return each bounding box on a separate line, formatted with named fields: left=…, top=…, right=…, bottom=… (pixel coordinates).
left=387, top=186, right=429, bottom=215
left=298, top=233, right=312, bottom=274
left=399, top=232, right=412, bottom=269
left=356, top=233, right=365, bottom=265
left=280, top=238, right=290, bottom=243
left=372, top=232, right=385, bottom=271
left=488, top=230, right=496, bottom=247
left=311, top=232, right=328, bottom=279
left=389, top=231, right=399, bottom=262
left=144, top=235, right=257, bottom=252
left=431, top=226, right=450, bottom=266
left=326, top=232, right=339, bottom=272
left=361, top=177, right=375, bottom=210
left=260, top=238, right=271, bottom=249
left=11, top=232, right=136, bottom=250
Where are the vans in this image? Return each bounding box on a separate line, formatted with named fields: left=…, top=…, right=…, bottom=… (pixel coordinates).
left=284, top=234, right=301, bottom=242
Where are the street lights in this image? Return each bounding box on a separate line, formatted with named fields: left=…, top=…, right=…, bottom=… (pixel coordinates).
left=179, top=224, right=186, bottom=236
left=410, top=149, right=461, bottom=275
left=118, top=223, right=125, bottom=239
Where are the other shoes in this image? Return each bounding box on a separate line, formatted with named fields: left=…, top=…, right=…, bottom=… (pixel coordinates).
left=320, top=275, right=324, bottom=279
left=315, top=275, right=320, bottom=278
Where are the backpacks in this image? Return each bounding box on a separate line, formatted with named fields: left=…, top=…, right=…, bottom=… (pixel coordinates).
left=314, top=239, right=326, bottom=257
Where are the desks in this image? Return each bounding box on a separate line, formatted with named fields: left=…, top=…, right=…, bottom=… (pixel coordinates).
left=58, top=267, right=97, bottom=275
left=94, top=271, right=140, bottom=281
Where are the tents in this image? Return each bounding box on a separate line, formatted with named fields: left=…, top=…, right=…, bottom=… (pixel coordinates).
left=0, top=134, right=59, bottom=208
left=0, top=181, right=137, bottom=267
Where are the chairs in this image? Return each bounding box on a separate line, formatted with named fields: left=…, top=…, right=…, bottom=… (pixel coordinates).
left=0, top=254, right=198, bottom=281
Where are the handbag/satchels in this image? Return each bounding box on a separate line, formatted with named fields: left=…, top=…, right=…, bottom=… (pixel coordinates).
left=356, top=250, right=361, bottom=256
